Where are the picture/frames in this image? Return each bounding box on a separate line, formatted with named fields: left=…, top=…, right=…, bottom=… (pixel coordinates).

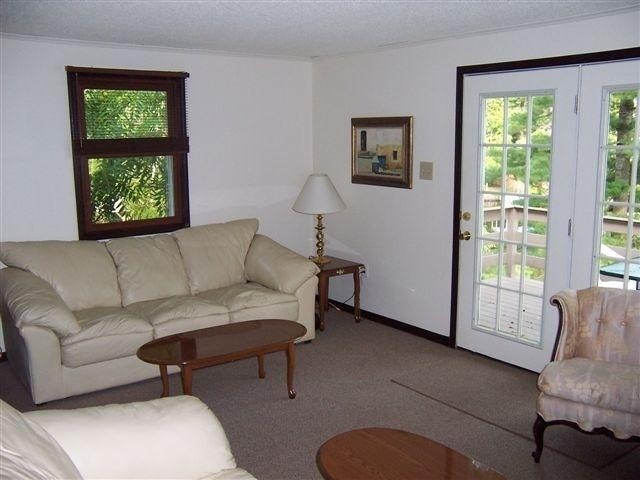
left=349, top=115, right=415, bottom=191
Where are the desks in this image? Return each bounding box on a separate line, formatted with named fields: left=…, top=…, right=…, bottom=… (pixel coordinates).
left=308, top=255, right=362, bottom=333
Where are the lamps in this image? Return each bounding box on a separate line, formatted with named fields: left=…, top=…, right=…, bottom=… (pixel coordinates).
left=292, top=172, right=348, bottom=265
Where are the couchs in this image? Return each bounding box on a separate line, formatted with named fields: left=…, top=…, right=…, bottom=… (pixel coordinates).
left=3, top=215, right=324, bottom=408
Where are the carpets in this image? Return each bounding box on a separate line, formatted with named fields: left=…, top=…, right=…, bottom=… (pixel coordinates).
left=388, top=347, right=640, bottom=473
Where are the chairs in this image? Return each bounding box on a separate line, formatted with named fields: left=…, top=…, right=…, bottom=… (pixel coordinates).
left=0, top=391, right=262, bottom=480
left=530, top=288, right=640, bottom=464
